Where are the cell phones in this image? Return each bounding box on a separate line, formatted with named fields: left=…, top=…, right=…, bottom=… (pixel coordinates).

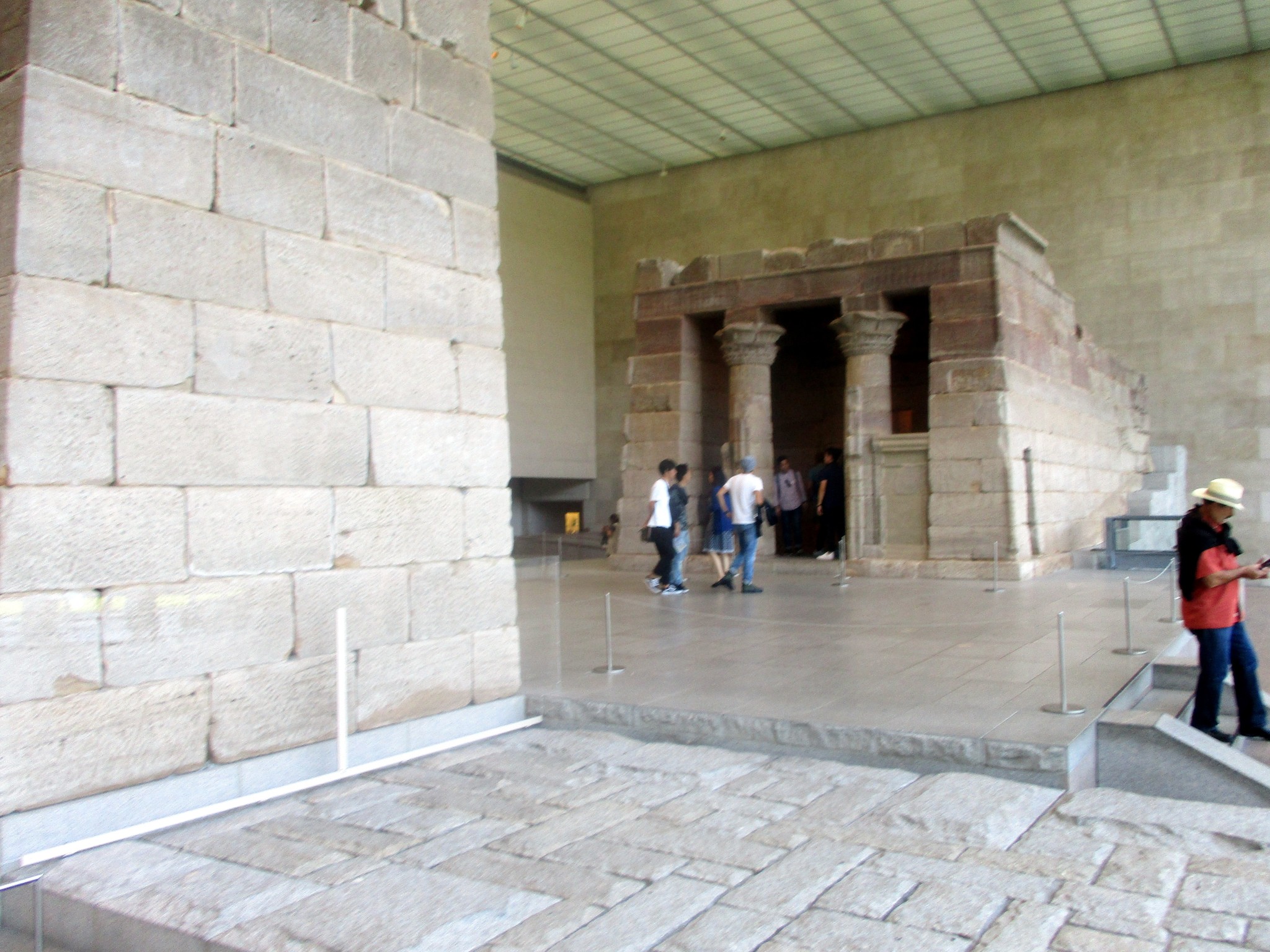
left=1261, top=559, right=1270, bottom=568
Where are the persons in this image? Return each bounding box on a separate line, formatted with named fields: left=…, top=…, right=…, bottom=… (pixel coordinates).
left=601, top=448, right=844, bottom=594
left=1175, top=478, right=1270, bottom=742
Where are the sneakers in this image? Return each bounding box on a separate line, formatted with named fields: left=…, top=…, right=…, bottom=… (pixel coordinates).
left=644, top=574, right=690, bottom=595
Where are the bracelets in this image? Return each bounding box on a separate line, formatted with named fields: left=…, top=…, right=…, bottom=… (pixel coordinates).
left=723, top=509, right=729, bottom=515
left=816, top=504, right=821, bottom=508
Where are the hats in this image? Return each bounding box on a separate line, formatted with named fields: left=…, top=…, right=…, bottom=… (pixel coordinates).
left=1192, top=478, right=1245, bottom=511
left=739, top=456, right=756, bottom=473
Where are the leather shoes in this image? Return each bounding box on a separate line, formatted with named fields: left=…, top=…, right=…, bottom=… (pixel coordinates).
left=1200, top=728, right=1234, bottom=742
left=1238, top=723, right=1270, bottom=740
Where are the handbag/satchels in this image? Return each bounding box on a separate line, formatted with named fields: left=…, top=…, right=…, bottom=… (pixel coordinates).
left=636, top=526, right=654, bottom=544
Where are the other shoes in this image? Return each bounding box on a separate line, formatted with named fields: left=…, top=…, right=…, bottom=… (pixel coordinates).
left=724, top=571, right=736, bottom=590
left=741, top=584, right=764, bottom=593
left=710, top=572, right=733, bottom=588
left=817, top=551, right=835, bottom=560
